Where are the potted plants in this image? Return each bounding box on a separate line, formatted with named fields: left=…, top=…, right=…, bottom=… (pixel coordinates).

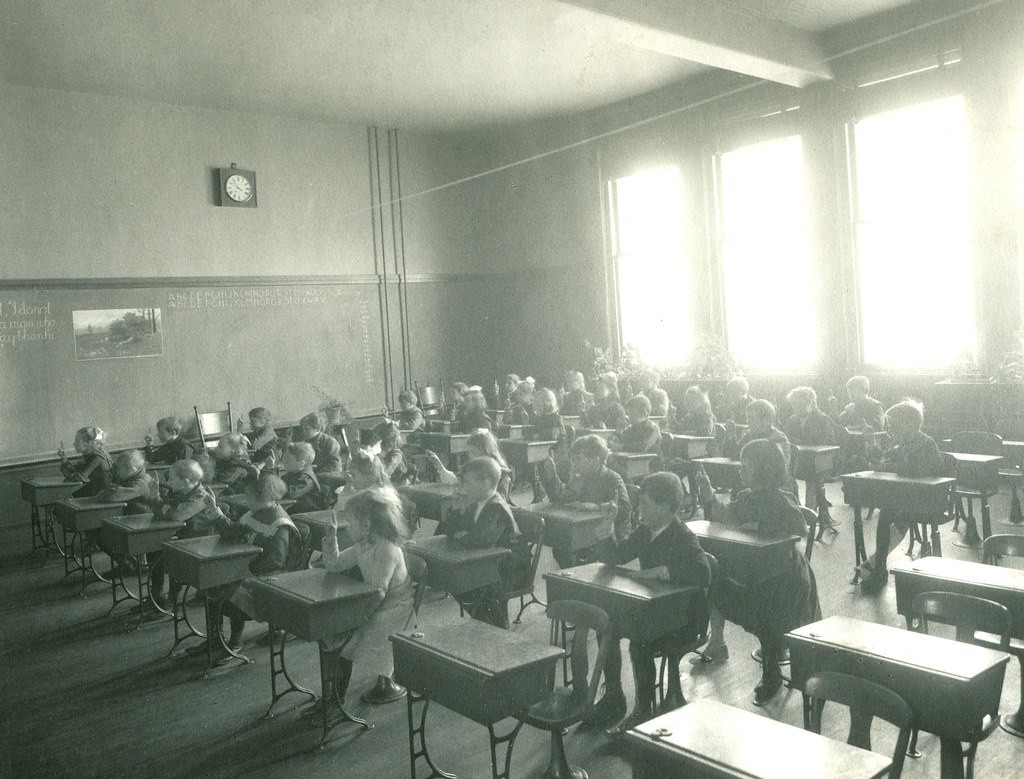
left=311, top=385, right=352, bottom=423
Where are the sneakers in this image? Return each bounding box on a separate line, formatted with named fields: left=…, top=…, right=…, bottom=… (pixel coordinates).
left=299, top=693, right=348, bottom=727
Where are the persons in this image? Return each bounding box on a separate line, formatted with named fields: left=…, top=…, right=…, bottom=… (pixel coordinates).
left=300, top=486, right=415, bottom=729
left=582, top=469, right=712, bottom=741
left=56, top=364, right=943, bottom=705
left=854, top=401, right=944, bottom=583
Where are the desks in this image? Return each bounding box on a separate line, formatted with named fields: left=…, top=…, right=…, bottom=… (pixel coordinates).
left=21, top=408, right=1024, bottom=779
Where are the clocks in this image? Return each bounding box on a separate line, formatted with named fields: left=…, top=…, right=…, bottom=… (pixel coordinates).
left=218, top=168, right=258, bottom=207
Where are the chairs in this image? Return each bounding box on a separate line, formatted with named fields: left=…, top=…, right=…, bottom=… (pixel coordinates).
left=904, top=591, right=1011, bottom=757
left=509, top=599, right=613, bottom=779
left=802, top=672, right=912, bottom=779
left=948, top=429, right=1006, bottom=550
left=194, top=401, right=233, bottom=449
left=978, top=534, right=1024, bottom=569
left=362, top=553, right=427, bottom=703
left=498, top=514, right=546, bottom=624
left=641, top=552, right=719, bottom=711
left=750, top=506, right=819, bottom=664
left=415, top=378, right=447, bottom=416
left=816, top=474, right=840, bottom=529
left=908, top=453, right=958, bottom=560
left=252, top=522, right=312, bottom=645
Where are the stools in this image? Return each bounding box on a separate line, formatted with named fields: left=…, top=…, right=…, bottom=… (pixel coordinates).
left=327, top=424, right=353, bottom=461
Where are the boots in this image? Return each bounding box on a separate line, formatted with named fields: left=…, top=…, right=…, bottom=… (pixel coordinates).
left=214, top=620, right=246, bottom=666
left=183, top=611, right=225, bottom=656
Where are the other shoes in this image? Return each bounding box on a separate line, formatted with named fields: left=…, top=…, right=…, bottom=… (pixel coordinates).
left=688, top=641, right=730, bottom=668
left=581, top=693, right=627, bottom=726
left=604, top=709, right=652, bottom=738
left=142, top=590, right=167, bottom=609
left=753, top=665, right=784, bottom=693
left=859, top=570, right=889, bottom=595
left=852, top=555, right=878, bottom=581
left=147, top=602, right=177, bottom=620
